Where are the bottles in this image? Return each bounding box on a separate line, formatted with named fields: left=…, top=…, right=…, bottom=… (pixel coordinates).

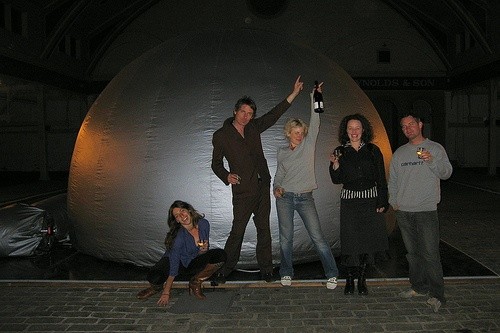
left=313, top=80, right=324, bottom=113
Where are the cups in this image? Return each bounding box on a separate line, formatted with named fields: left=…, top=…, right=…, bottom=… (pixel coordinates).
left=278, top=189, right=285, bottom=198
left=233, top=173, right=241, bottom=185
left=417, top=147, right=426, bottom=159
left=197, top=239, right=207, bottom=250
left=333, top=149, right=340, bottom=161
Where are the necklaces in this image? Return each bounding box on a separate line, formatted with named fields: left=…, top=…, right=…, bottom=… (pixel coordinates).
left=359, top=142, right=362, bottom=149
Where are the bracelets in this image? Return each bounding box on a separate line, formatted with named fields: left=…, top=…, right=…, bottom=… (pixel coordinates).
left=161, top=293, right=169, bottom=296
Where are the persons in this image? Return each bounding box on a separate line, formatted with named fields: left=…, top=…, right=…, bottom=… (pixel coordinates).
left=137, top=200, right=225, bottom=305
left=212, top=76, right=303, bottom=281
left=388, top=112, right=452, bottom=304
left=328, top=113, right=389, bottom=296
left=273, top=81, right=338, bottom=290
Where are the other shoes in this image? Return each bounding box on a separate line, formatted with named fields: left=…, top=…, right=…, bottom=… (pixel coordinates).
left=189, top=284, right=205, bottom=301
left=135, top=285, right=163, bottom=299
left=357, top=274, right=368, bottom=294
left=345, top=274, right=355, bottom=294
left=213, top=276, right=225, bottom=285
left=281, top=275, right=293, bottom=287
left=327, top=277, right=338, bottom=289
left=263, top=274, right=274, bottom=282
